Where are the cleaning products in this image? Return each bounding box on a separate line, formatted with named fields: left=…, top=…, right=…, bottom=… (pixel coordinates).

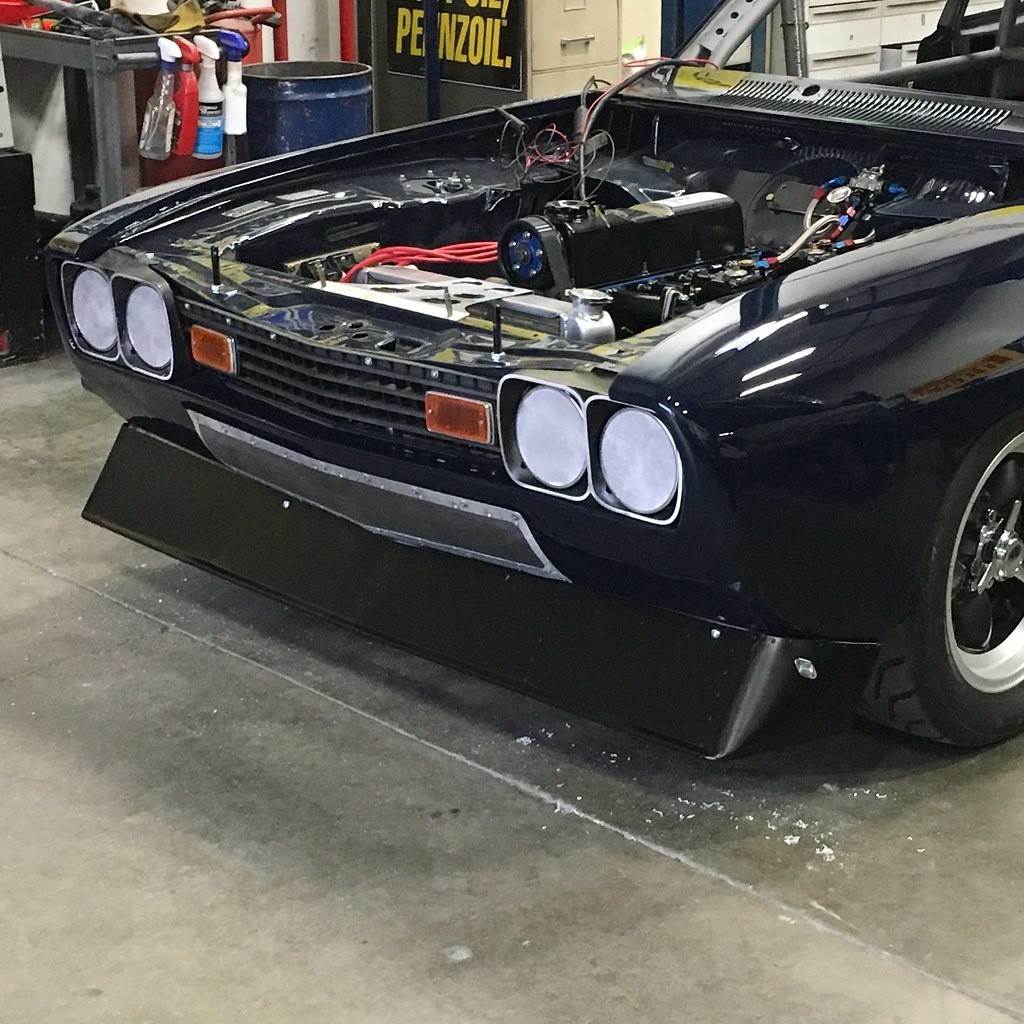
left=188, top=37, right=226, bottom=162
left=217, top=29, right=249, bottom=140
left=135, top=35, right=183, bottom=164
left=166, top=33, right=202, bottom=158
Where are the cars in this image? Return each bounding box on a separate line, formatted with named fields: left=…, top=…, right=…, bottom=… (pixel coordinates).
left=40, top=4, right=1024, bottom=776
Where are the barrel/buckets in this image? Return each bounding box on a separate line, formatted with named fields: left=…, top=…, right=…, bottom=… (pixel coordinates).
left=239, top=59, right=376, bottom=163
